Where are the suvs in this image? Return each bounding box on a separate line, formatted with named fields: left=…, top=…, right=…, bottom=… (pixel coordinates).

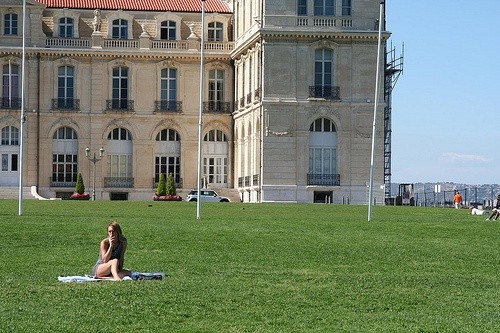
left=186, top=190, right=231, bottom=202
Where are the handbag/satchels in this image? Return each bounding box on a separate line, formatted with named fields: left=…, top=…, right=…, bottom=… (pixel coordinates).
left=131, top=271, right=162, bottom=281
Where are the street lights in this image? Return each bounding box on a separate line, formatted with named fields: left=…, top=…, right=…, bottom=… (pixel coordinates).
left=85, top=147, right=105, bottom=201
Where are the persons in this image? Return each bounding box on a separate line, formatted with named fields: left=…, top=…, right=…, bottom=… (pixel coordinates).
left=94, top=222, right=132, bottom=281
left=486, top=195, right=500, bottom=220
left=453, top=192, right=462, bottom=210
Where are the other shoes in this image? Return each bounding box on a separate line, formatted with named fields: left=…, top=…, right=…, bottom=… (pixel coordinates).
left=493, top=219, right=496, bottom=222
left=486, top=218, right=490, bottom=221
